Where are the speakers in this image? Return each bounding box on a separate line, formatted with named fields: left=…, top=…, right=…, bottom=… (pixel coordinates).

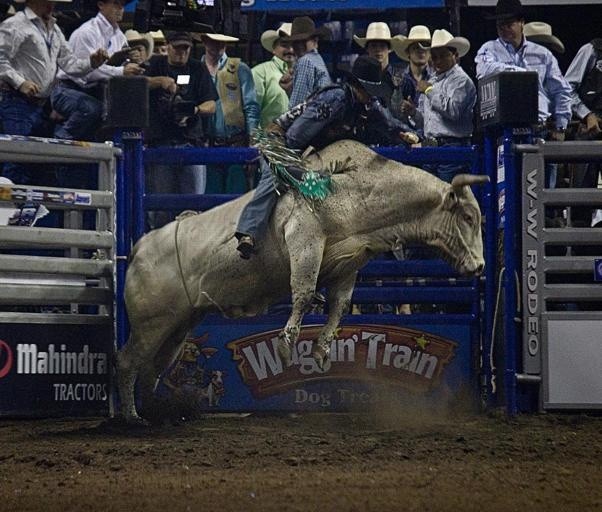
left=476, top=72, right=539, bottom=131
left=97, top=76, right=149, bottom=129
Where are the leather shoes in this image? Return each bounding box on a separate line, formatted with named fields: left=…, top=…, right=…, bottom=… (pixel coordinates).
left=235, top=235, right=253, bottom=259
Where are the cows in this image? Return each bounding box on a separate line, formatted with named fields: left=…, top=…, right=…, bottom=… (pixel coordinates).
left=111, top=139, right=491, bottom=430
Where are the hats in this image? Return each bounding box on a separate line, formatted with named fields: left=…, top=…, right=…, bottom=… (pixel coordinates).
left=524, top=20, right=566, bottom=54
left=124, top=26, right=241, bottom=62
left=262, top=15, right=472, bottom=59
left=482, top=0, right=529, bottom=21
left=337, top=54, right=393, bottom=99
